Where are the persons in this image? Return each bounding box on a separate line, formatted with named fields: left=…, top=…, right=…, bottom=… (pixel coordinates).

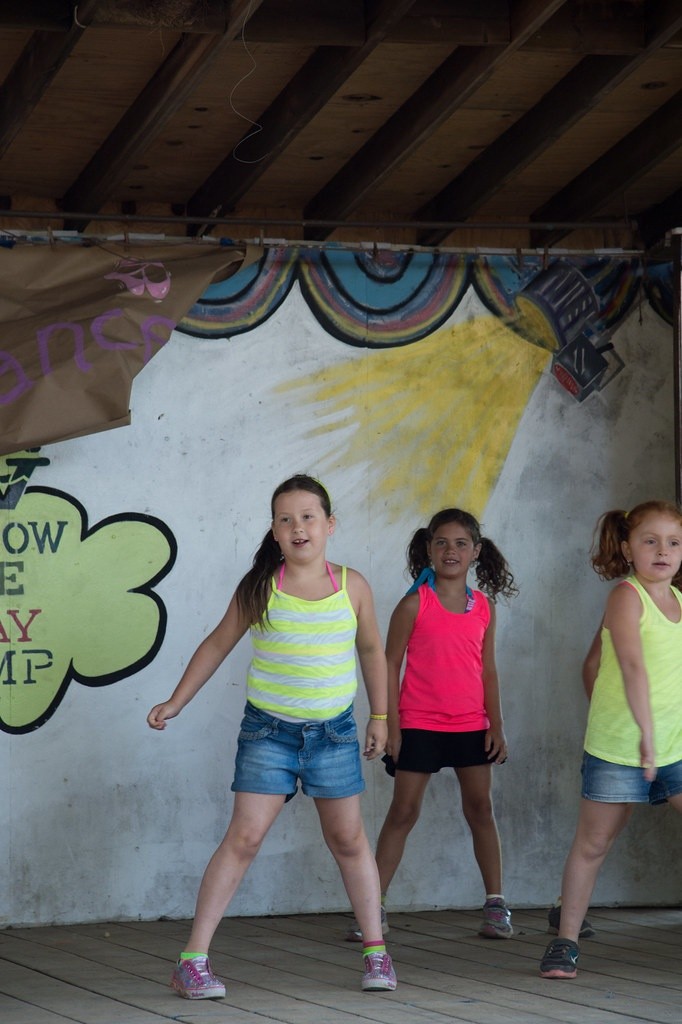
left=146, top=475, right=398, bottom=1000
left=538, top=501, right=681, bottom=981
left=347, top=508, right=519, bottom=939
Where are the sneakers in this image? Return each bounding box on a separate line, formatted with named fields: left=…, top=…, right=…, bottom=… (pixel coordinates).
left=361, top=952, right=398, bottom=991
left=546, top=903, right=596, bottom=938
left=167, top=954, right=226, bottom=1000
left=344, top=903, right=390, bottom=941
left=479, top=897, right=514, bottom=939
left=538, top=937, right=581, bottom=979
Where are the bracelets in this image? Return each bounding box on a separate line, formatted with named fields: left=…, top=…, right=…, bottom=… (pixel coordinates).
left=370, top=714, right=388, bottom=720
